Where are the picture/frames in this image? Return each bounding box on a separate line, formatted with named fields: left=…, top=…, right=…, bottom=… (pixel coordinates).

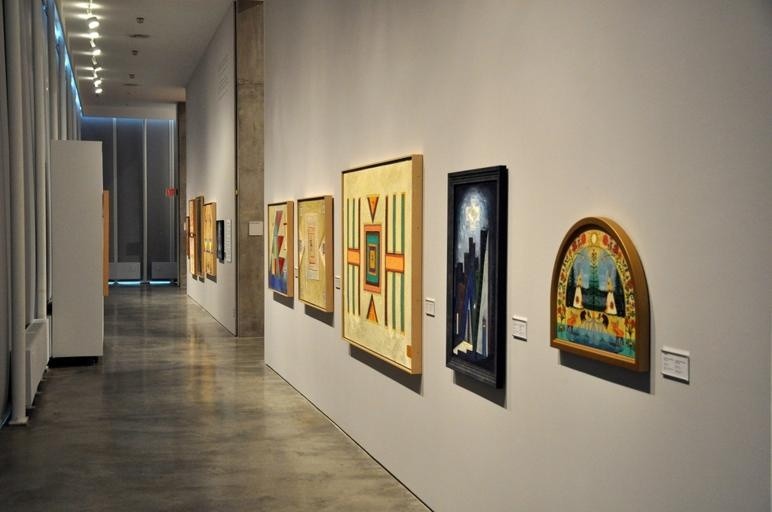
left=337, top=155, right=427, bottom=380
left=547, top=214, right=649, bottom=376
left=295, top=194, right=336, bottom=315
left=436, top=163, right=512, bottom=390
left=188, top=195, right=230, bottom=279
left=267, top=200, right=294, bottom=301
